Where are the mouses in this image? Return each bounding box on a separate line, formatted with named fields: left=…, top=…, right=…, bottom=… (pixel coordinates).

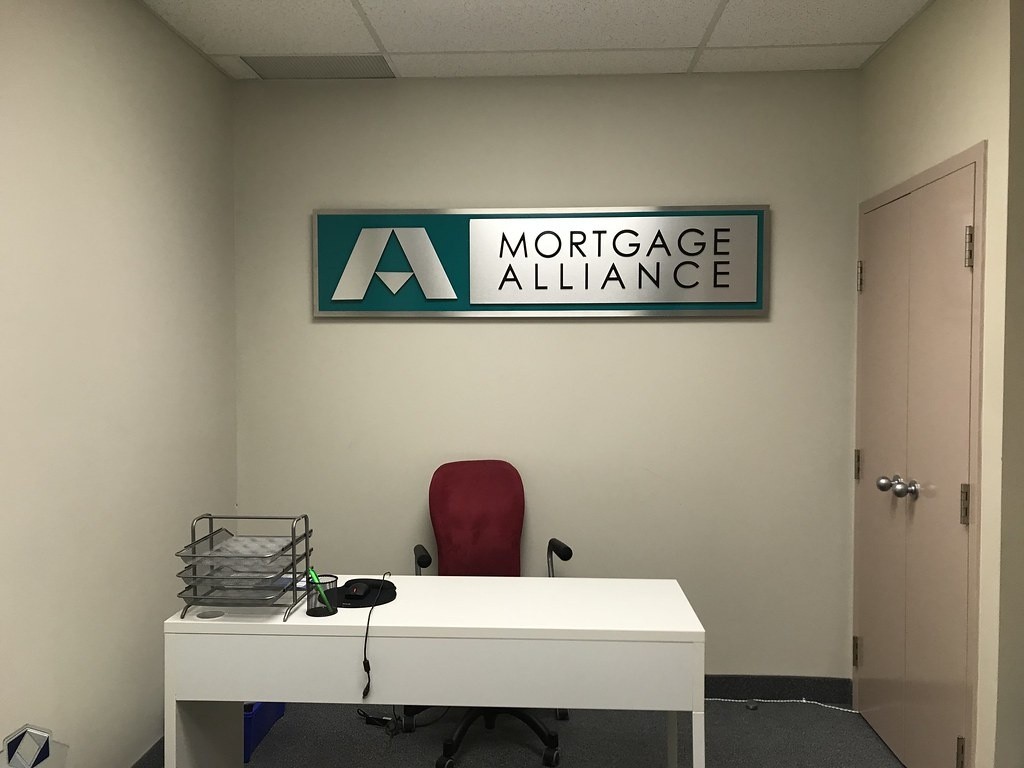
left=344, top=583, right=370, bottom=601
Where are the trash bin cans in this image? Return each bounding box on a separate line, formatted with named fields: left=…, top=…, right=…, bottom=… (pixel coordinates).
left=245, top=702, right=287, bottom=762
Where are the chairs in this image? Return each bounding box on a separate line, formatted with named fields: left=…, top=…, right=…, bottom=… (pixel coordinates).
left=413, top=459, right=573, bottom=768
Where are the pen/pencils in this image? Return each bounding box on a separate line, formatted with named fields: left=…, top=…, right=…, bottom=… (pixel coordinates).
left=305, top=565, right=332, bottom=613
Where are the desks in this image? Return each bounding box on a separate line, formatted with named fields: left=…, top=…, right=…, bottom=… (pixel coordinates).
left=164, top=574, right=705, bottom=768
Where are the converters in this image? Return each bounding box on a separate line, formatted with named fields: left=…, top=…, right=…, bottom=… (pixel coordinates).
left=366, top=714, right=393, bottom=726
